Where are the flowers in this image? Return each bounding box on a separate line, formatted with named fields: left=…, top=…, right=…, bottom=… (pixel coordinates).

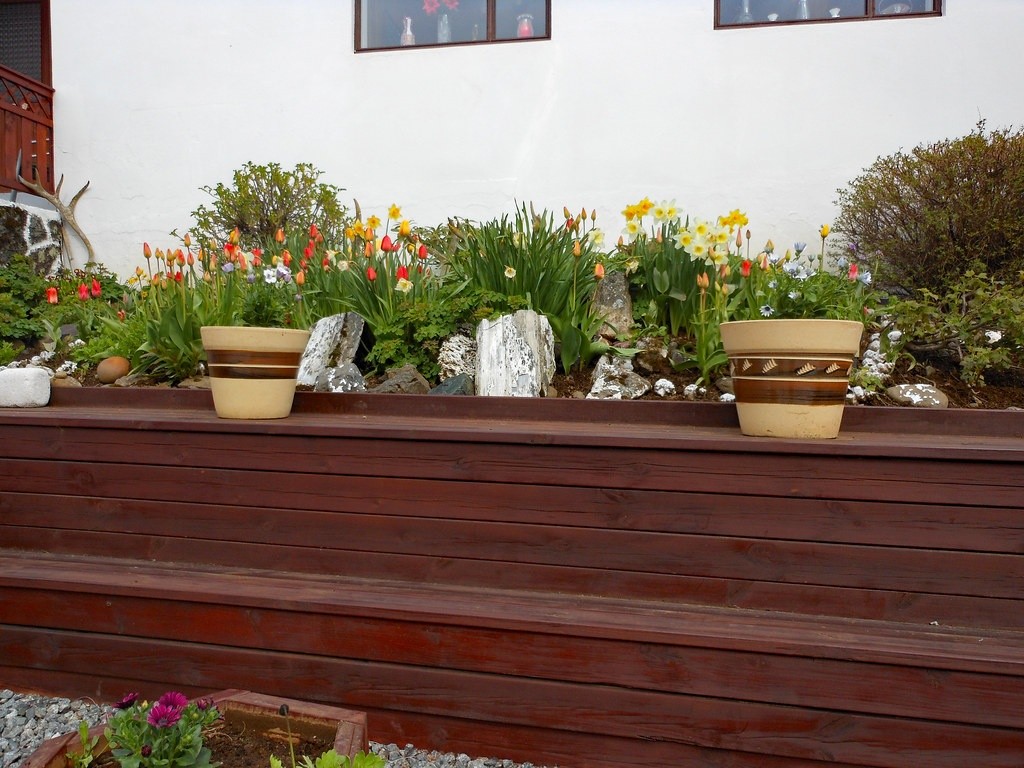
left=219, top=262, right=305, bottom=330
left=59, top=689, right=387, bottom=766
left=731, top=241, right=875, bottom=326
left=421, top=0, right=459, bottom=16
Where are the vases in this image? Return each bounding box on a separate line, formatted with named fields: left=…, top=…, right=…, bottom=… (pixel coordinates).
left=200, top=325, right=309, bottom=419
left=438, top=14, right=451, bottom=43
left=720, top=319, right=863, bottom=438
left=18, top=690, right=369, bottom=767
